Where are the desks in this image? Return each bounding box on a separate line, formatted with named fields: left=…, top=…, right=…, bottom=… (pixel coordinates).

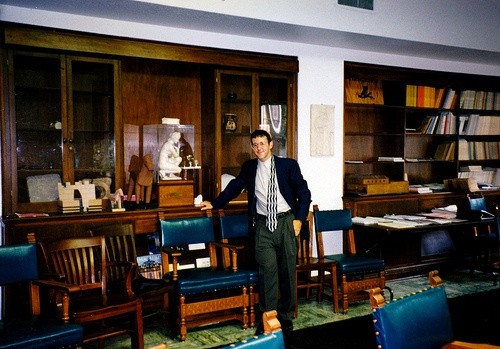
left=351, top=210, right=497, bottom=286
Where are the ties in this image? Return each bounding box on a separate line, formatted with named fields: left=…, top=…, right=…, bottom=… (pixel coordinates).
left=267, top=155, right=278, bottom=232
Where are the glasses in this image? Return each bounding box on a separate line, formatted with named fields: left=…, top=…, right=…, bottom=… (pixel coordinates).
left=252, top=142, right=266, bottom=148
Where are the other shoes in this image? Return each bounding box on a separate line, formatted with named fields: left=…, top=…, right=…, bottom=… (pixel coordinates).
left=281, top=320, right=293, bottom=330
left=255, top=323, right=264, bottom=336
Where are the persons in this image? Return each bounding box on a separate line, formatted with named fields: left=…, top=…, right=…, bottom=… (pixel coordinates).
left=200, top=130, right=311, bottom=335
left=158, top=131, right=182, bottom=180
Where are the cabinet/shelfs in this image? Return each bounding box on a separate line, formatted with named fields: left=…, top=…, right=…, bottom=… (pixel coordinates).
left=0, top=20, right=298, bottom=307
left=343, top=60, right=500, bottom=282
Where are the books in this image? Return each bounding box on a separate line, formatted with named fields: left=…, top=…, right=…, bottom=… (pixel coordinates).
left=405, top=85, right=500, bottom=109
left=345, top=174, right=409, bottom=197
left=405, top=138, right=500, bottom=163
left=405, top=112, right=500, bottom=135
left=378, top=156, right=404, bottom=162
left=345, top=161, right=363, bottom=163
left=408, top=165, right=500, bottom=194
left=351, top=208, right=467, bottom=229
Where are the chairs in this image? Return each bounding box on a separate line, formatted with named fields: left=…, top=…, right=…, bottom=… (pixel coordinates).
left=0, top=191, right=500, bottom=349
